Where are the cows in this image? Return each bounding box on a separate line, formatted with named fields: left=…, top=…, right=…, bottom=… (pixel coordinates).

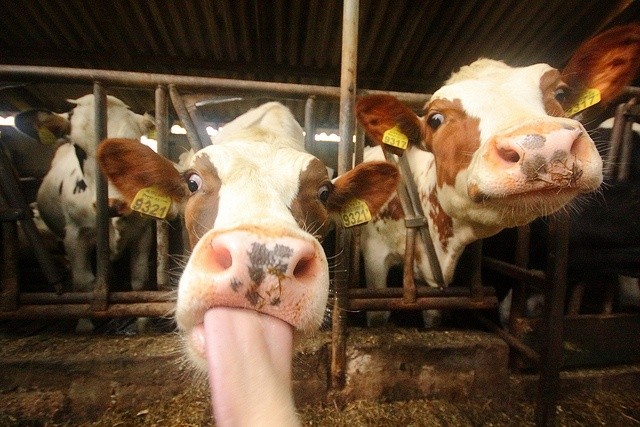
left=355, top=23, right=636, bottom=333
left=97, top=100, right=402, bottom=427
left=15, top=93, right=174, bottom=339
